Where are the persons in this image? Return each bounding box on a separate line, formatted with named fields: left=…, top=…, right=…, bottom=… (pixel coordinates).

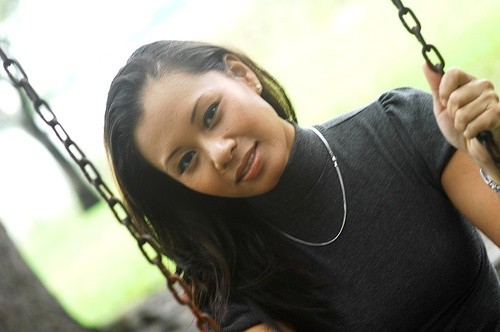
left=101, top=36, right=500, bottom=332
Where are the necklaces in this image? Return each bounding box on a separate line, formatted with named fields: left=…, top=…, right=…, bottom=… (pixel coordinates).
left=254, top=125, right=347, bottom=248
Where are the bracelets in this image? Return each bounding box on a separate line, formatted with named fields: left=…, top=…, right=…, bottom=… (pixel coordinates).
left=477, top=167, right=500, bottom=193
